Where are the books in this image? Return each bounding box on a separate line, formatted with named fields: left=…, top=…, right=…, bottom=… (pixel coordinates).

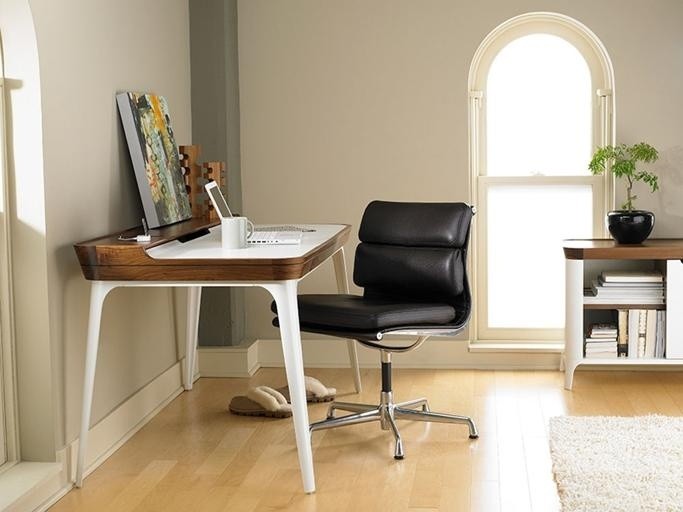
left=583, top=270, right=667, bottom=360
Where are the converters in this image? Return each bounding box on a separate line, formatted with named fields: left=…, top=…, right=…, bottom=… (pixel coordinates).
left=136, top=235, right=151, bottom=242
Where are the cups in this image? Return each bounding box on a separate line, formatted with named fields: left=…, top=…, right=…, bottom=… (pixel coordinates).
left=221, top=216, right=255, bottom=250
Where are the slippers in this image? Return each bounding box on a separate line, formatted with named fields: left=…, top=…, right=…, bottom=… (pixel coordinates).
left=274, top=376, right=336, bottom=405
left=228, top=386, right=294, bottom=419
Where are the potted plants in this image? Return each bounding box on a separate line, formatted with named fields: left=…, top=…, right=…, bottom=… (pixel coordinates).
left=588, top=139, right=661, bottom=243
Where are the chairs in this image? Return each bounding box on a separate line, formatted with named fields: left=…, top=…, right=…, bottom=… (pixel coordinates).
left=268, top=197, right=480, bottom=461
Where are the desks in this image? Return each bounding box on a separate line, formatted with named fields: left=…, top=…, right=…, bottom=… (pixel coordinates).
left=64, top=213, right=360, bottom=495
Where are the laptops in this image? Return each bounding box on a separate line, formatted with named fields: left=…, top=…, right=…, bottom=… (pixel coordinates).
left=205, top=181, right=302, bottom=244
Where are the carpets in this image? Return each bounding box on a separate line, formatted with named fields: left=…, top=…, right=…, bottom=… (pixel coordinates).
left=540, top=408, right=683, bottom=511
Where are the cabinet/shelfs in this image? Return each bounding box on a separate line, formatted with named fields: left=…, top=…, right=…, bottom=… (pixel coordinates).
left=556, top=233, right=682, bottom=393
